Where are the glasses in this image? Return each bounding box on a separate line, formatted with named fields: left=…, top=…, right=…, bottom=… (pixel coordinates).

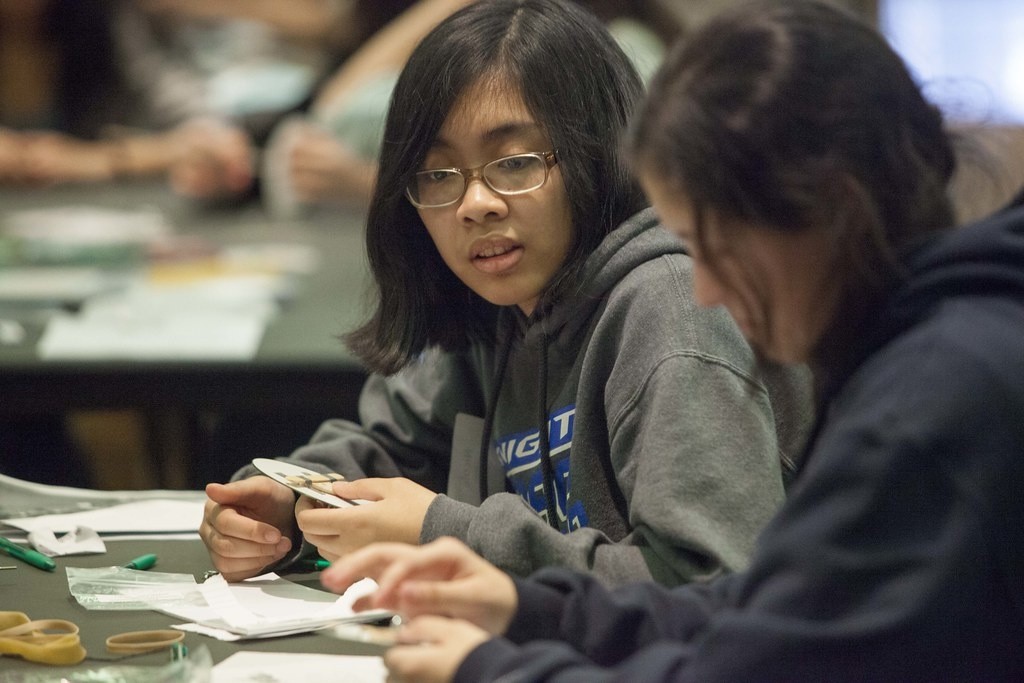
left=404, top=145, right=571, bottom=208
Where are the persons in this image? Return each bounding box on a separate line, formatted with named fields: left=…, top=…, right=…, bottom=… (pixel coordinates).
left=0, top=0, right=688, bottom=490
left=199, top=0, right=820, bottom=592
left=323, top=0, right=1024, bottom=683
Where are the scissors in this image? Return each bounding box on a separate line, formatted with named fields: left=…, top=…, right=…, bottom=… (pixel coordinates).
left=279, top=471, right=345, bottom=495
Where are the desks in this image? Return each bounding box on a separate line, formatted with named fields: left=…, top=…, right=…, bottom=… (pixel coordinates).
left=0, top=177, right=379, bottom=471
left=0, top=484, right=398, bottom=683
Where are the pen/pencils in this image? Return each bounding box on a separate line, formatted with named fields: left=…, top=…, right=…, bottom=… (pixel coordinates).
left=0, top=537, right=57, bottom=570
left=295, top=559, right=332, bottom=571
left=325, top=622, right=400, bottom=647
left=122, top=553, right=157, bottom=570
